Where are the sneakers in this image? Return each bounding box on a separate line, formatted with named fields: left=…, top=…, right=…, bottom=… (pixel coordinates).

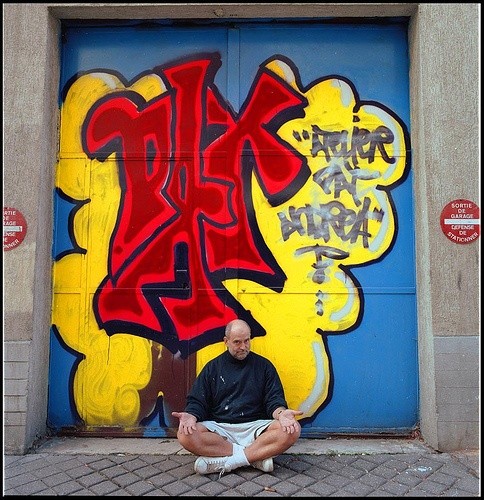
left=251, top=457, right=273, bottom=472
left=194, top=456, right=231, bottom=475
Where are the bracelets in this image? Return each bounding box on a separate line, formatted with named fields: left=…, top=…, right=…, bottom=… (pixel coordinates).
left=277, top=410, right=283, bottom=414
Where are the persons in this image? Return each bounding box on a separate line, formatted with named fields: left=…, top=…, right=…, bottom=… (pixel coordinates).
left=171, top=319, right=302, bottom=477
